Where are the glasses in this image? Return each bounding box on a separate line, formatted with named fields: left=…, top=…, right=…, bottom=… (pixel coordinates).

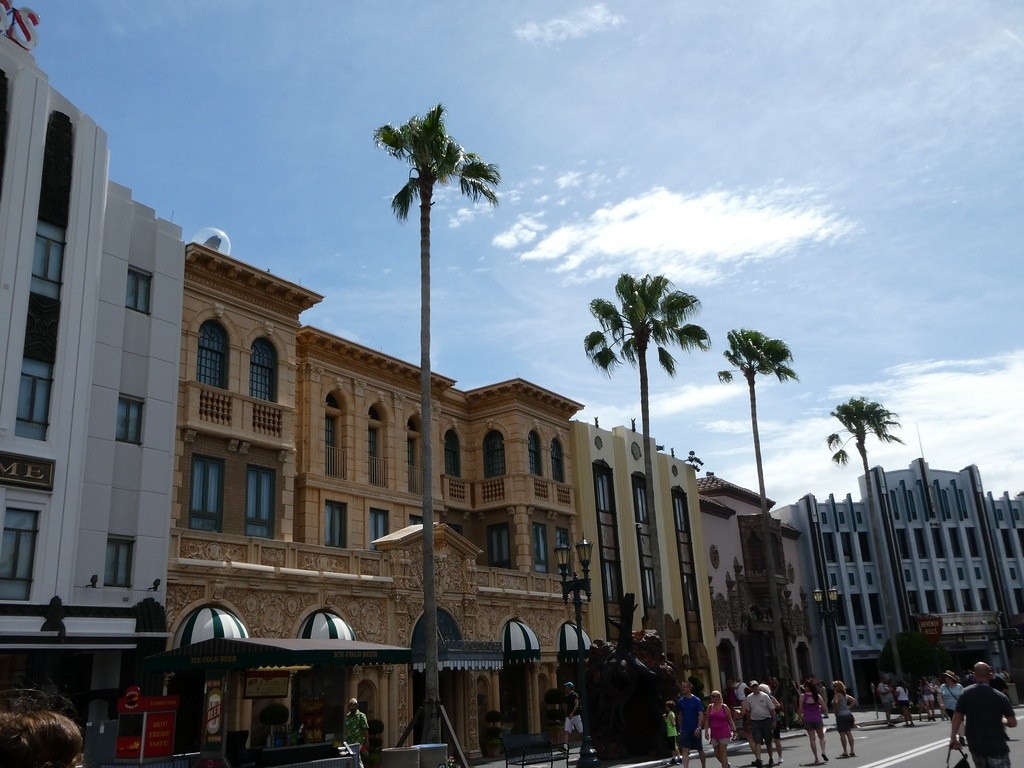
left=711, top=695, right=719, bottom=698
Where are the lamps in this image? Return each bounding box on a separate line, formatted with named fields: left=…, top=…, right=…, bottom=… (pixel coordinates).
left=149, top=579, right=160, bottom=592
left=86, top=575, right=98, bottom=588
left=681, top=653, right=692, bottom=672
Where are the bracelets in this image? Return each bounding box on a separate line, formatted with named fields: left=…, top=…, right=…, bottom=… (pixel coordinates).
left=697, top=726, right=701, bottom=732
left=733, top=731, right=736, bottom=732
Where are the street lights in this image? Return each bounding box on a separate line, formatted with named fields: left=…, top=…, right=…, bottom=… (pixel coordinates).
left=813, top=586, right=852, bottom=699
left=552, top=531, right=599, bottom=768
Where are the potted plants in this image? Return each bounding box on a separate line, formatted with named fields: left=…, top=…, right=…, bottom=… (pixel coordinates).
left=483, top=710, right=506, bottom=757
left=543, top=688, right=569, bottom=745
left=688, top=675, right=709, bottom=729
left=367, top=719, right=384, bottom=768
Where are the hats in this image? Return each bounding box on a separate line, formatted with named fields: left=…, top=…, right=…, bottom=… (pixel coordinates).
left=564, top=682, right=574, bottom=688
left=750, top=680, right=758, bottom=687
left=943, top=670, right=957, bottom=681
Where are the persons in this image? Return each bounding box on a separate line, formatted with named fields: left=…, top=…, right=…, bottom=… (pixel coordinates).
left=740, top=680, right=783, bottom=768
left=677, top=680, right=706, bottom=768
left=877, top=675, right=895, bottom=727
left=895, top=680, right=915, bottom=727
left=799, top=680, right=829, bottom=763
left=725, top=678, right=742, bottom=705
left=818, top=680, right=829, bottom=718
left=832, top=681, right=856, bottom=757
left=963, top=666, right=1010, bottom=693
left=663, top=700, right=682, bottom=766
left=937, top=670, right=965, bottom=746
left=916, top=675, right=949, bottom=722
left=949, top=661, right=1018, bottom=768
left=704, top=690, right=737, bottom=768
left=559, top=682, right=583, bottom=753
left=344, top=698, right=369, bottom=768
left=733, top=678, right=749, bottom=706
left=0, top=710, right=82, bottom=768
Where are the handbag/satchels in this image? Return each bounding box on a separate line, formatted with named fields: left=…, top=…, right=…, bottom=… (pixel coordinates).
left=774, top=710, right=784, bottom=721
left=946, top=746, right=970, bottom=768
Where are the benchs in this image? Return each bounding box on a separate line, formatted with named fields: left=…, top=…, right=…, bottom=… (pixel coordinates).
left=499, top=733, right=570, bottom=768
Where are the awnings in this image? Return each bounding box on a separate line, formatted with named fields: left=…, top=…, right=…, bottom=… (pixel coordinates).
left=145, top=637, right=413, bottom=753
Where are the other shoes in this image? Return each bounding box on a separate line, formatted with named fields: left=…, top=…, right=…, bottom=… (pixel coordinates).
left=752, top=759, right=763, bottom=768
left=959, top=737, right=966, bottom=746
left=849, top=753, right=855, bottom=757
left=768, top=759, right=773, bottom=768
left=887, top=723, right=896, bottom=728
left=822, top=754, right=828, bottom=761
left=779, top=756, right=783, bottom=762
left=559, top=748, right=567, bottom=754
left=676, top=759, right=682, bottom=765
left=840, top=753, right=848, bottom=757
left=667, top=759, right=677, bottom=766
